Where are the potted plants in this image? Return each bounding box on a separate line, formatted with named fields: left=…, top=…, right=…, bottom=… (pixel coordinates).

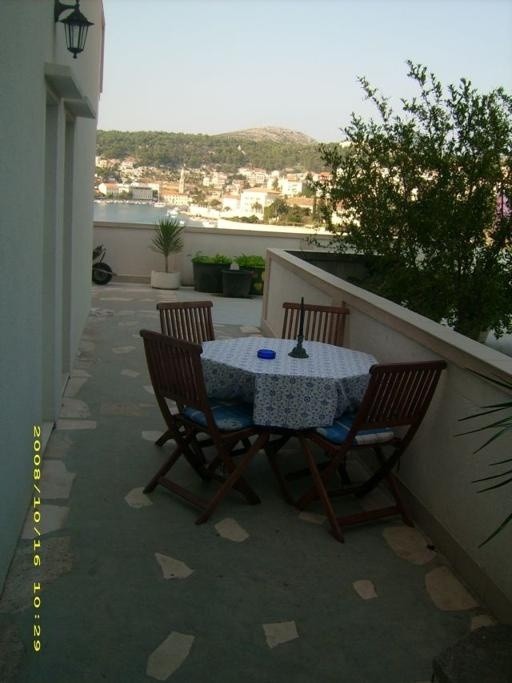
left=147, top=218, right=186, bottom=290
left=190, top=251, right=233, bottom=293
left=232, top=253, right=265, bottom=296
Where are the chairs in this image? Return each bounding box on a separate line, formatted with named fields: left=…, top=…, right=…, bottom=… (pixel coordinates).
left=282, top=302, right=350, bottom=347
left=291, top=359, right=447, bottom=543
left=140, top=329, right=271, bottom=526
left=155, top=301, right=217, bottom=447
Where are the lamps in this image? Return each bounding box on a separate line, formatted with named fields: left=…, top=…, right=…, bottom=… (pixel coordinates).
left=53, top=0, right=95, bottom=58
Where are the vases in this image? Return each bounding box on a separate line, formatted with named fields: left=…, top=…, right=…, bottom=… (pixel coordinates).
left=220, top=269, right=253, bottom=298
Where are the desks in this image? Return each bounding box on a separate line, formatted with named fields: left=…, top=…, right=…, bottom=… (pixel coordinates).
left=200, top=337, right=379, bottom=471
